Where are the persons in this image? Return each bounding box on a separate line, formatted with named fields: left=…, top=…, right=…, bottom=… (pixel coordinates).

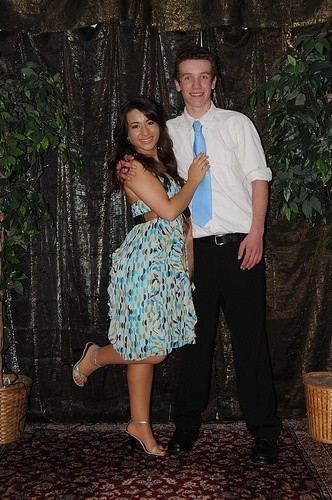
left=72, top=96, right=211, bottom=456
left=116, top=48, right=283, bottom=465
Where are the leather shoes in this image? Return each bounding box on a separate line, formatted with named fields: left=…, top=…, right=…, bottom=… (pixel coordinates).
left=168, top=429, right=198, bottom=453
left=251, top=438, right=278, bottom=465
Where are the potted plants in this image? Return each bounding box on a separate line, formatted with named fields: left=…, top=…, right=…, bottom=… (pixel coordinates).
left=0, top=58, right=82, bottom=444
left=249, top=25, right=332, bottom=442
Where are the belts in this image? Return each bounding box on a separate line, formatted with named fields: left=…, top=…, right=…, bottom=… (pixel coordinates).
left=194, top=233, right=248, bottom=246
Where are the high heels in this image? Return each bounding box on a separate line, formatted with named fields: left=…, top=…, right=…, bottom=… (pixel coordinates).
left=124, top=420, right=166, bottom=457
left=73, top=341, right=104, bottom=387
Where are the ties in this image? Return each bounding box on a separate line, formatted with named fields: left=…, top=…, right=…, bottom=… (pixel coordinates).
left=192, top=121, right=213, bottom=228
